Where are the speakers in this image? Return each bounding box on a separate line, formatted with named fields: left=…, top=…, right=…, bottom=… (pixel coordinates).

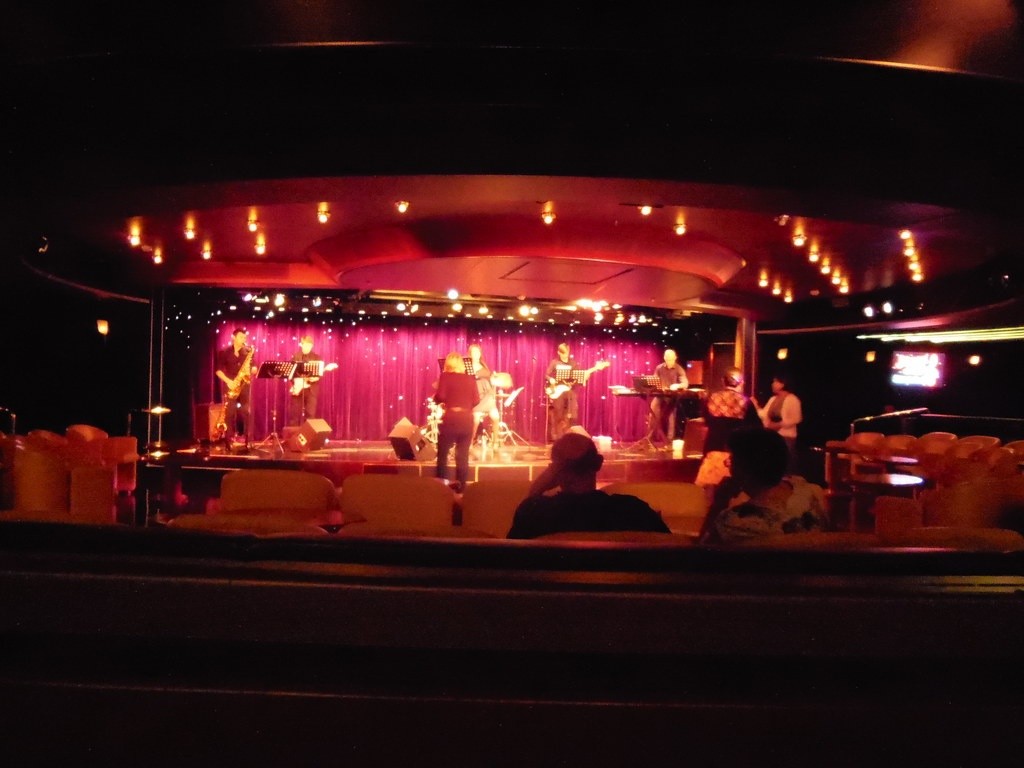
left=288, top=418, right=333, bottom=451
left=389, top=424, right=438, bottom=462
left=195, top=402, right=226, bottom=442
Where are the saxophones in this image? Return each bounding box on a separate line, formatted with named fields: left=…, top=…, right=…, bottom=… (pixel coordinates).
left=226, top=342, right=256, bottom=401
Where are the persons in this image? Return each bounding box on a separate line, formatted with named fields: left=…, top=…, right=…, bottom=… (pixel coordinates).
left=505, top=432, right=673, bottom=542
left=464, top=344, right=502, bottom=455
left=650, top=349, right=689, bottom=441
left=703, top=367, right=763, bottom=455
left=699, top=426, right=833, bottom=542
left=213, top=326, right=255, bottom=452
left=543, top=341, right=590, bottom=441
left=749, top=375, right=803, bottom=476
left=430, top=351, right=481, bottom=494
left=285, top=333, right=320, bottom=426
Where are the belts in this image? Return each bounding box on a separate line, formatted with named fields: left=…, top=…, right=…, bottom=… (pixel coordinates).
left=447, top=407, right=471, bottom=413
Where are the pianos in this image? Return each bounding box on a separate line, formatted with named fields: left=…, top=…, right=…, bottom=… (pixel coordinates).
left=608, top=385, right=709, bottom=456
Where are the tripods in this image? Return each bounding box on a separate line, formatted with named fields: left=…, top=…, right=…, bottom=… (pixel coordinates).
left=254, top=360, right=296, bottom=453
left=626, top=375, right=662, bottom=453
left=490, top=394, right=532, bottom=449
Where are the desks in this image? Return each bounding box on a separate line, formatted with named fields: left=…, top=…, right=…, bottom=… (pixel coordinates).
left=810, top=445, right=859, bottom=492
left=841, top=473, right=926, bottom=529
left=863, top=454, right=918, bottom=475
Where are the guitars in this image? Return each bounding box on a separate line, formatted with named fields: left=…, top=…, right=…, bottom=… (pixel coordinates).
left=543, top=360, right=611, bottom=400
left=289, top=363, right=339, bottom=397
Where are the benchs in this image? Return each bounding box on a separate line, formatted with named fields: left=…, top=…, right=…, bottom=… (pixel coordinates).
left=0, top=520, right=1024, bottom=768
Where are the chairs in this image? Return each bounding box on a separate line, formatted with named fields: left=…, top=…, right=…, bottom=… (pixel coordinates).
left=1, top=424, right=1024, bottom=550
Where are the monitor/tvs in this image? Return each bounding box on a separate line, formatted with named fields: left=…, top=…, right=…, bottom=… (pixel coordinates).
left=890, top=351, right=945, bottom=388
